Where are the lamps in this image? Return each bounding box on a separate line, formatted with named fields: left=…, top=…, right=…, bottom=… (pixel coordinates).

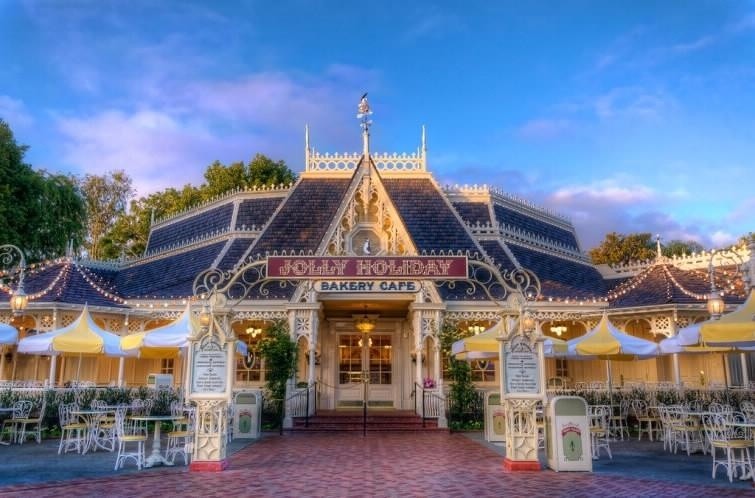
left=355, top=305, right=376, bottom=334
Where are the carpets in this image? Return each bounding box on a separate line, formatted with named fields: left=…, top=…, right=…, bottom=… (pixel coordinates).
left=462, top=431, right=755, bottom=490
left=1, top=438, right=261, bottom=485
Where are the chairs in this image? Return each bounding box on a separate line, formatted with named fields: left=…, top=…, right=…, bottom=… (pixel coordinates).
left=1, top=399, right=196, bottom=472
left=588, top=399, right=755, bottom=483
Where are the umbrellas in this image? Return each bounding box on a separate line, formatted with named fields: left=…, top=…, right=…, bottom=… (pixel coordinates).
left=120, top=301, right=248, bottom=404
left=554, top=309, right=660, bottom=439
left=452, top=313, right=569, bottom=361
left=18, top=301, right=139, bottom=386
left=660, top=284, right=755, bottom=406
left=0, top=323, right=20, bottom=345
